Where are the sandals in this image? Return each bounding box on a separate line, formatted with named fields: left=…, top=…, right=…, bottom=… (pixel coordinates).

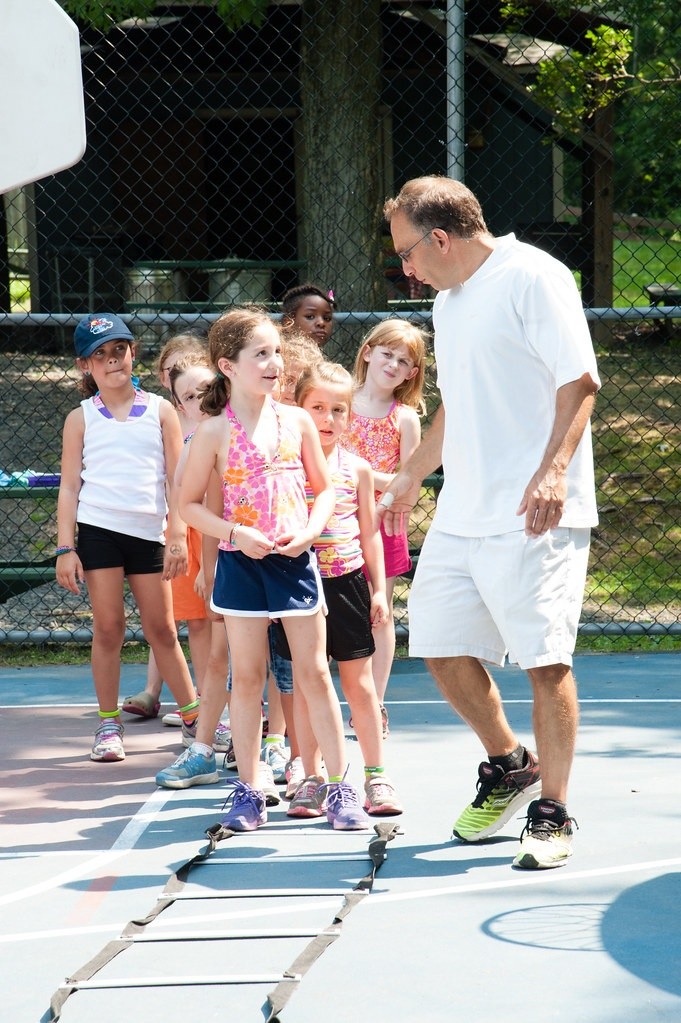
left=122, top=691, right=161, bottom=717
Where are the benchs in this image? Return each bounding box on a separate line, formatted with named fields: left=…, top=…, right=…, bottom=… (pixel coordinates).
left=644, top=281, right=680, bottom=336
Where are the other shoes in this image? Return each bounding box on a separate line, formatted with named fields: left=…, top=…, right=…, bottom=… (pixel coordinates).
left=262, top=720, right=288, bottom=737
left=162, top=709, right=182, bottom=726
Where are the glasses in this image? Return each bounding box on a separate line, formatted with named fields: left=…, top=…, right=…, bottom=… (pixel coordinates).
left=398, top=226, right=452, bottom=263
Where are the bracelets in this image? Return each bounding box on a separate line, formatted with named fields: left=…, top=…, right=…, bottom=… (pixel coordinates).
left=231, top=522, right=243, bottom=547
left=230, top=528, right=234, bottom=543
left=55, top=549, right=71, bottom=556
left=54, top=546, right=76, bottom=551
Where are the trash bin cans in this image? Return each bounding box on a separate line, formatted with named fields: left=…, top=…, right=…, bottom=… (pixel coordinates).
left=208, top=259, right=272, bottom=314
left=122, top=267, right=186, bottom=356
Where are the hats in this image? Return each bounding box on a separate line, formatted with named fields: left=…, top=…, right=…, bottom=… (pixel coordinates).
left=74, top=313, right=135, bottom=358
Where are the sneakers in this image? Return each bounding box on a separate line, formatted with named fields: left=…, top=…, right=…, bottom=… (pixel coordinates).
left=91, top=717, right=126, bottom=761
left=513, top=798, right=579, bottom=868
left=364, top=775, right=403, bottom=815
left=261, top=741, right=289, bottom=782
left=380, top=705, right=389, bottom=739
left=287, top=776, right=327, bottom=816
left=285, top=757, right=305, bottom=798
left=181, top=716, right=233, bottom=751
left=220, top=776, right=267, bottom=832
left=259, top=762, right=280, bottom=803
left=453, top=747, right=542, bottom=841
left=155, top=746, right=219, bottom=788
left=222, top=734, right=237, bottom=769
left=318, top=763, right=369, bottom=830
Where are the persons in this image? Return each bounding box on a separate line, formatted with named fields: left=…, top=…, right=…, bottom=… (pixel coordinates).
left=180, top=305, right=368, bottom=833
left=121, top=284, right=427, bottom=816
left=54, top=313, right=232, bottom=763
left=381, top=175, right=603, bottom=867
